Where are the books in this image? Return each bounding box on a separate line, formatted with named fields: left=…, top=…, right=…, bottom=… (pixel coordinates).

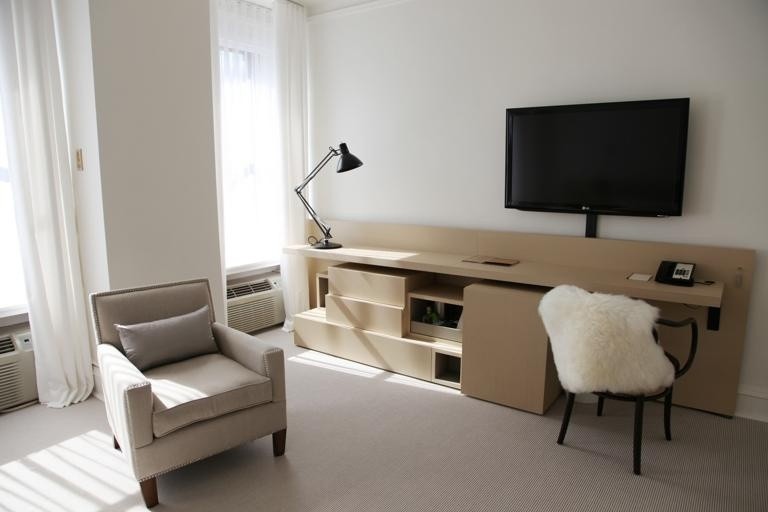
left=461, top=252, right=520, bottom=268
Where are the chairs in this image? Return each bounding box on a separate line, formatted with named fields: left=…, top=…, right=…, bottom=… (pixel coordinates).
left=89, top=279, right=288, bottom=508
left=539, top=283, right=698, bottom=474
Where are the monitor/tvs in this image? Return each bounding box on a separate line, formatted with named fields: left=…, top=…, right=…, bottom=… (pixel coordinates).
left=504, top=97, right=690, bottom=217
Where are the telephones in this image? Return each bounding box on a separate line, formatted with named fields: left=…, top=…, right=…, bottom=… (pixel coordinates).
left=655, top=260, right=697, bottom=287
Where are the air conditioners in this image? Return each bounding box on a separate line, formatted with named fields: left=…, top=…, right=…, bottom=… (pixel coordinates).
left=0, top=328, right=40, bottom=415
left=225, top=275, right=286, bottom=335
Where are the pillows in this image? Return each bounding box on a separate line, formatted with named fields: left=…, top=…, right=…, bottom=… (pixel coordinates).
left=112, top=304, right=218, bottom=370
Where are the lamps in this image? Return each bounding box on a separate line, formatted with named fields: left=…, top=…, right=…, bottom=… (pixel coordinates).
left=292, top=143, right=364, bottom=251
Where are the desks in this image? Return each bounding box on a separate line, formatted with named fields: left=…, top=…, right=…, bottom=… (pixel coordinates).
left=293, top=216, right=757, bottom=416
